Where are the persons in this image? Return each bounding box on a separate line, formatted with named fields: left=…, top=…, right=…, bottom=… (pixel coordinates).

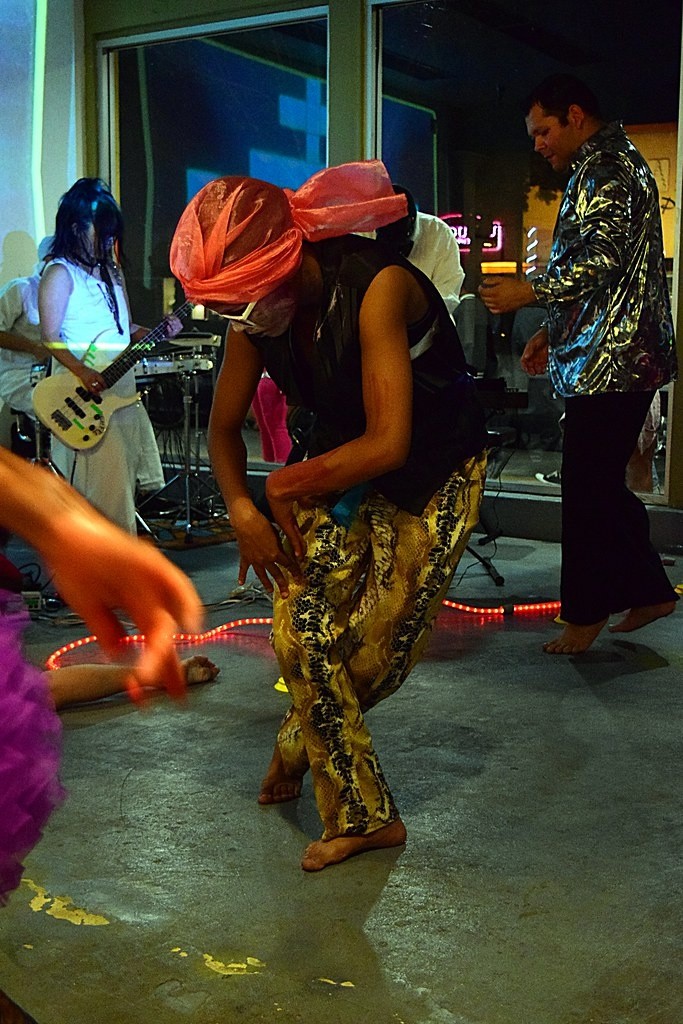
left=475, top=71, right=679, bottom=653
left=344, top=184, right=465, bottom=313
left=251, top=370, right=292, bottom=466
left=171, top=160, right=490, bottom=873
left=534, top=388, right=660, bottom=493
left=0, top=444, right=206, bottom=1024
left=0, top=176, right=184, bottom=548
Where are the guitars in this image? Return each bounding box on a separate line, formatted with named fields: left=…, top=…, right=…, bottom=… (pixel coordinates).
left=31, top=299, right=196, bottom=451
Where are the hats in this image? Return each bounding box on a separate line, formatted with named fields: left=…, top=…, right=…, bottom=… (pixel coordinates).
left=39, top=236, right=56, bottom=262
left=375, top=184, right=417, bottom=244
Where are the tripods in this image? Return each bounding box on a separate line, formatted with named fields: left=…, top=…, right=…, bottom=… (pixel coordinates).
left=133, top=376, right=225, bottom=542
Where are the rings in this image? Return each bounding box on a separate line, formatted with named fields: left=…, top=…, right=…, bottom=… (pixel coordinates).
left=90, top=381, right=99, bottom=387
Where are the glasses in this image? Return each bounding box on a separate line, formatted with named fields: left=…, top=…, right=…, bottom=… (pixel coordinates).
left=207, top=301, right=258, bottom=331
